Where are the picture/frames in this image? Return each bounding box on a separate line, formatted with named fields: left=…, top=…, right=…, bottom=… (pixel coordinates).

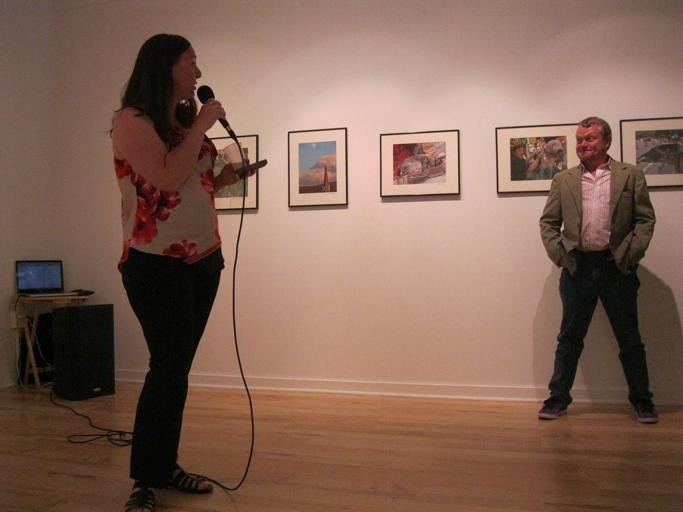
left=495, top=123, right=584, bottom=193
left=287, top=127, right=348, bottom=207
left=208, top=134, right=258, bottom=211
left=619, top=116, right=683, bottom=188
left=380, top=129, right=461, bottom=199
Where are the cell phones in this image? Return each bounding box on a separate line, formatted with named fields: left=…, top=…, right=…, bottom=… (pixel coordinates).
left=236, top=159, right=267, bottom=179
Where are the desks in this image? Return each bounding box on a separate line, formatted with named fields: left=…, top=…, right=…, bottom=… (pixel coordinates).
left=12, top=291, right=91, bottom=393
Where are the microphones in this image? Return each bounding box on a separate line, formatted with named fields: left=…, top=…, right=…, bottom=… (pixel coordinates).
left=197, top=86, right=239, bottom=145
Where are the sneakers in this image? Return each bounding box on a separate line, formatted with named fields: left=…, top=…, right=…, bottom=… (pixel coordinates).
left=634, top=399, right=658, bottom=423
left=538, top=395, right=569, bottom=420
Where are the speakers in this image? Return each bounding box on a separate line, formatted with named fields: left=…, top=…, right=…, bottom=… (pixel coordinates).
left=53, top=304, right=114, bottom=401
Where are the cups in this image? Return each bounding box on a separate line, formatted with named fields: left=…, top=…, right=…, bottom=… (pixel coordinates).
left=219, top=139, right=246, bottom=174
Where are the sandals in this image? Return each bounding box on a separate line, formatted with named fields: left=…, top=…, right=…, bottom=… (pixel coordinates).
left=164, top=463, right=215, bottom=495
left=123, top=480, right=158, bottom=512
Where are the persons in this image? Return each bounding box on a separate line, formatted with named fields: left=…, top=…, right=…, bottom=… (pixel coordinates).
left=536, top=115, right=661, bottom=424
left=110, top=33, right=258, bottom=512
left=510, top=144, right=533, bottom=180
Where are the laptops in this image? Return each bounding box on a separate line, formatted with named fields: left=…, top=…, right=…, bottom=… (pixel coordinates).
left=15, top=260, right=78, bottom=297
